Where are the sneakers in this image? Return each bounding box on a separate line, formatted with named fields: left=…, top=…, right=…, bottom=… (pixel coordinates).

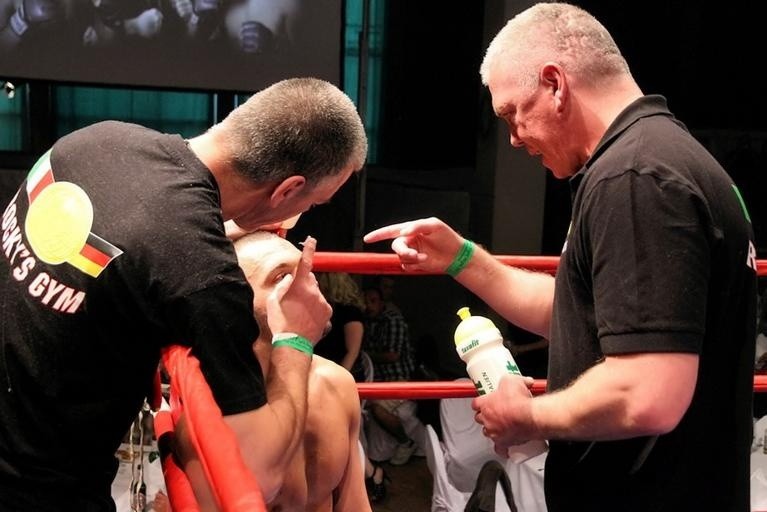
left=390, top=435, right=418, bottom=465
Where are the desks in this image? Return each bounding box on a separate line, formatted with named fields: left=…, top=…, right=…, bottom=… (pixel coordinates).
left=508, top=438, right=550, bottom=512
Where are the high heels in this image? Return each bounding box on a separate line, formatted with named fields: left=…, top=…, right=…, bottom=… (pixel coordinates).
left=366, top=466, right=392, bottom=502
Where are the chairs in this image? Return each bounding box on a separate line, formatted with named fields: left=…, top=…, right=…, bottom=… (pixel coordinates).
left=361, top=350, right=374, bottom=409
left=425, top=424, right=511, bottom=512
left=425, top=377, right=509, bottom=493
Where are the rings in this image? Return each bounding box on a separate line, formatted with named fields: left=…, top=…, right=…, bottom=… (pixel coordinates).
left=483, top=428, right=490, bottom=437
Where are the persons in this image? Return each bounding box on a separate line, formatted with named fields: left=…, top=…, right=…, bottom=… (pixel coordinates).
left=1, top=72, right=367, bottom=512
left=0, top=0, right=330, bottom=96
left=359, top=0, right=758, bottom=510
left=173, top=229, right=373, bottom=512
left=752, top=303, right=766, bottom=419
left=310, top=269, right=393, bottom=504
left=359, top=282, right=418, bottom=468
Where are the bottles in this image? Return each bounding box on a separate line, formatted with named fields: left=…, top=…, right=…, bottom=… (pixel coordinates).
left=450, top=307, right=551, bottom=464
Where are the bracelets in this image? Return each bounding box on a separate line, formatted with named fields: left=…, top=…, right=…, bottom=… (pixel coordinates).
left=444, top=238, right=475, bottom=281
left=268, top=331, right=315, bottom=356
